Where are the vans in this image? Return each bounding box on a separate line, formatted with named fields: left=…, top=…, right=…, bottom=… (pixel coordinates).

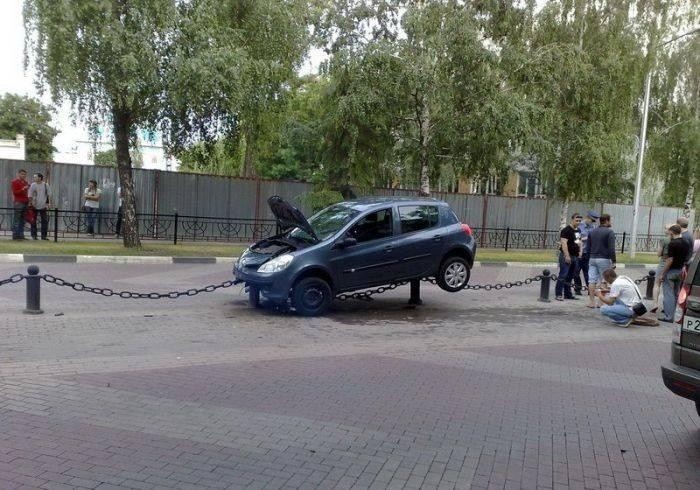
left=660, top=252, right=700, bottom=419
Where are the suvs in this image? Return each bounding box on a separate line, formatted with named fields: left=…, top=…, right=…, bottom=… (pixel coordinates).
left=233, top=193, right=478, bottom=317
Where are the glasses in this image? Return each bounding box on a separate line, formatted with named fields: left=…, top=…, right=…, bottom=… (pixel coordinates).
left=574, top=219, right=582, bottom=223
left=589, top=217, right=597, bottom=223
left=576, top=215, right=583, bottom=218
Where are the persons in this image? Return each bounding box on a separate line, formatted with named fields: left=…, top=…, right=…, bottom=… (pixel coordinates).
left=657, top=224, right=687, bottom=323
left=686, top=226, right=700, bottom=265
left=555, top=213, right=583, bottom=301
left=83, top=180, right=102, bottom=236
left=649, top=224, right=674, bottom=314
left=28, top=173, right=52, bottom=241
left=585, top=214, right=617, bottom=309
left=594, top=269, right=643, bottom=328
left=676, top=217, right=694, bottom=263
left=10, top=169, right=30, bottom=240
left=113, top=187, right=122, bottom=238
left=574, top=210, right=601, bottom=296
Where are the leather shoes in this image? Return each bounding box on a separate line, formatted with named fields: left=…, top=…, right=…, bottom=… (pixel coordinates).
left=658, top=315, right=675, bottom=323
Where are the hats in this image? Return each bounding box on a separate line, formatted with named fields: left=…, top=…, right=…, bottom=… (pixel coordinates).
left=587, top=208, right=601, bottom=219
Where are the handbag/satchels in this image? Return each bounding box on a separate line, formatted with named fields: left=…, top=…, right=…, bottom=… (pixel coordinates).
left=81, top=189, right=90, bottom=212
left=622, top=274, right=648, bottom=317
left=23, top=204, right=37, bottom=226
left=44, top=183, right=50, bottom=204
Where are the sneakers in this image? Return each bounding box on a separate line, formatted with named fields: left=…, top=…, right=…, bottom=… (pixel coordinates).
left=615, top=318, right=635, bottom=329
left=568, top=295, right=581, bottom=301
left=611, top=320, right=617, bottom=325
left=556, top=294, right=564, bottom=301
